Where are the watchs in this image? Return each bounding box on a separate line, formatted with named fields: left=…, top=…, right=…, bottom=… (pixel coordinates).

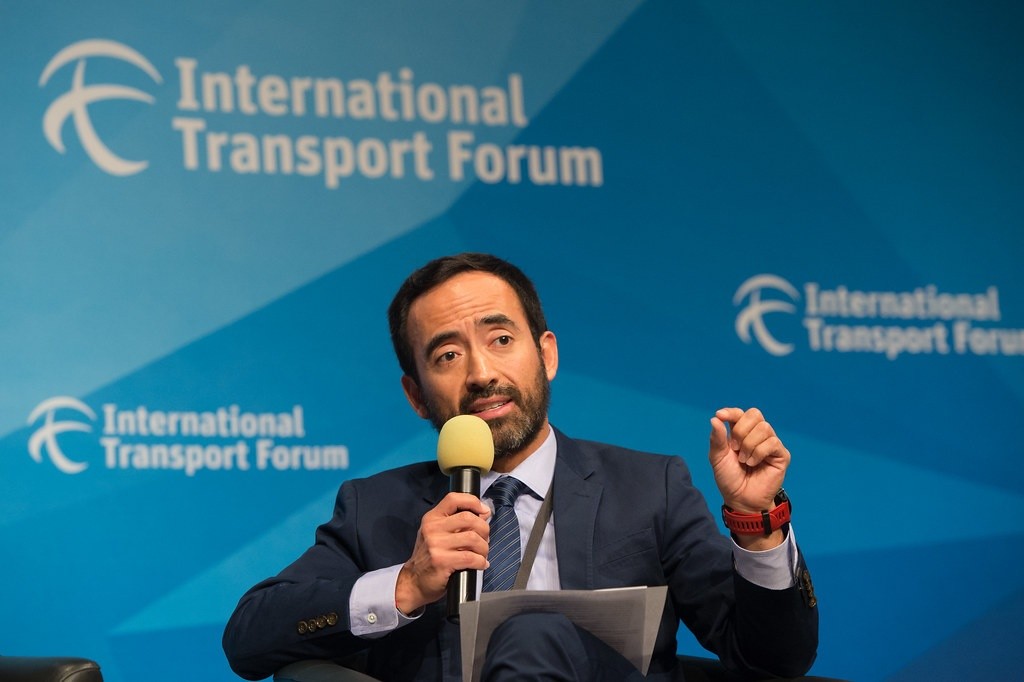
left=721, top=487, right=793, bottom=535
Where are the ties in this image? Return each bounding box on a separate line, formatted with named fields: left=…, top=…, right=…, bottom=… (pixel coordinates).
left=480, top=474, right=545, bottom=594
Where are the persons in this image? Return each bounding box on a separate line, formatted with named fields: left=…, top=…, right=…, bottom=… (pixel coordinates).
left=220, top=253, right=821, bottom=682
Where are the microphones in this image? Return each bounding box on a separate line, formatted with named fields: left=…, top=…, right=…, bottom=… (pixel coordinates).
left=437, top=414, right=494, bottom=626
